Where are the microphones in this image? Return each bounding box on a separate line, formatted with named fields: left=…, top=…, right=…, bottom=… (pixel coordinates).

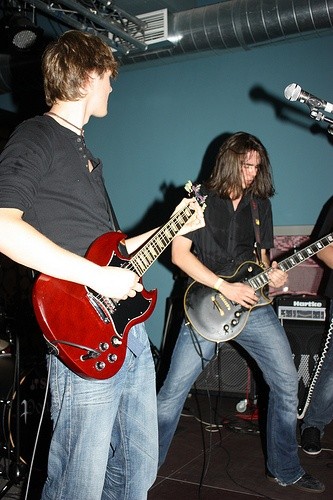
left=284, top=83, right=333, bottom=115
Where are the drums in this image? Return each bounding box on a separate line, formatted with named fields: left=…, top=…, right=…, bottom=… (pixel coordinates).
left=0, top=340, right=16, bottom=406
left=2, top=359, right=54, bottom=474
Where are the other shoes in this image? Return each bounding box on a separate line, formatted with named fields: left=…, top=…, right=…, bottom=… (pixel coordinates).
left=267, top=467, right=326, bottom=493
left=301, top=427, right=321, bottom=455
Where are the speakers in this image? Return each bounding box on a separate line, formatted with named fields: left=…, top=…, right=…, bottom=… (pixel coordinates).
left=281, top=323, right=328, bottom=415
left=189, top=340, right=262, bottom=400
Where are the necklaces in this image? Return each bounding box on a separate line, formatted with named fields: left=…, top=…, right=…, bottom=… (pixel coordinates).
left=48, top=112, right=82, bottom=131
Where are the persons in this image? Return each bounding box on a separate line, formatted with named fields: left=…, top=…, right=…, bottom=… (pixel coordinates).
left=0, top=30, right=207, bottom=500
left=296, top=196, right=333, bottom=456
left=155, top=130, right=326, bottom=493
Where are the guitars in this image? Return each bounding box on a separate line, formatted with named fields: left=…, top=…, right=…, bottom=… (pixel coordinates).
left=31, top=179, right=209, bottom=382
left=183, top=231, right=333, bottom=343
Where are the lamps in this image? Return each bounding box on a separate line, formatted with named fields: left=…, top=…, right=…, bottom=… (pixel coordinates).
left=3, top=16, right=42, bottom=53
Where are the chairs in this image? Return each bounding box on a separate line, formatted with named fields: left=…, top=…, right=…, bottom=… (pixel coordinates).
left=0, top=317, right=26, bottom=485
